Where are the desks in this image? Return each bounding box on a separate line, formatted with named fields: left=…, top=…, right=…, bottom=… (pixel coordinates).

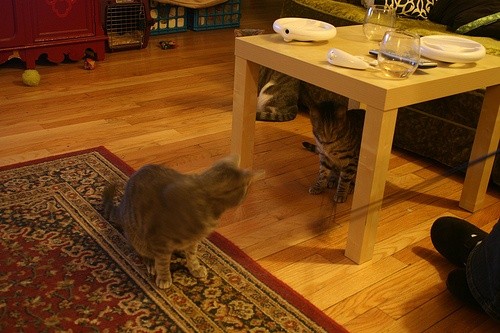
left=231, top=24, right=500, bottom=264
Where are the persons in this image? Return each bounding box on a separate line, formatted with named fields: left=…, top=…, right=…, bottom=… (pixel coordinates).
left=431, top=217, right=500, bottom=315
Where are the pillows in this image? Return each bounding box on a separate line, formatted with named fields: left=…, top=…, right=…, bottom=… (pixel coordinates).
left=384, top=0, right=435, bottom=20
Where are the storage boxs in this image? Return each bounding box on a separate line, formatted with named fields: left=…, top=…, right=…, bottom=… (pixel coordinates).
left=148, top=0, right=241, bottom=35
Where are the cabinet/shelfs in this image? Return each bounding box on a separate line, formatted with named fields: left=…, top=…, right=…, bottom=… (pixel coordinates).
left=0, top=0, right=109, bottom=70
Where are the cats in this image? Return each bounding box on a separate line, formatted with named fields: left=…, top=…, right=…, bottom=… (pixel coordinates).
left=236, top=28, right=366, bottom=203
left=102, top=154, right=265, bottom=289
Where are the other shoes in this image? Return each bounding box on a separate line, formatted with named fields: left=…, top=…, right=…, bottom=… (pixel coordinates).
left=447, top=268, right=486, bottom=309
left=430, top=217, right=489, bottom=265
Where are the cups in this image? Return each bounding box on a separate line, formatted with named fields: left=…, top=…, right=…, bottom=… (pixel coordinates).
left=377, top=30, right=420, bottom=79
left=362, top=4, right=396, bottom=43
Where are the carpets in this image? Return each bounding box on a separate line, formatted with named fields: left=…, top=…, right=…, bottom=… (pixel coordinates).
left=0, top=146, right=350, bottom=333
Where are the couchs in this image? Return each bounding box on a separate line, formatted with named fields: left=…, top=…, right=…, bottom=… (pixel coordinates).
left=278, top=0, right=500, bottom=187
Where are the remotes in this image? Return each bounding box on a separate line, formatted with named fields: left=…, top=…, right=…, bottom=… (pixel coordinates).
left=371, top=48, right=438, bottom=69
